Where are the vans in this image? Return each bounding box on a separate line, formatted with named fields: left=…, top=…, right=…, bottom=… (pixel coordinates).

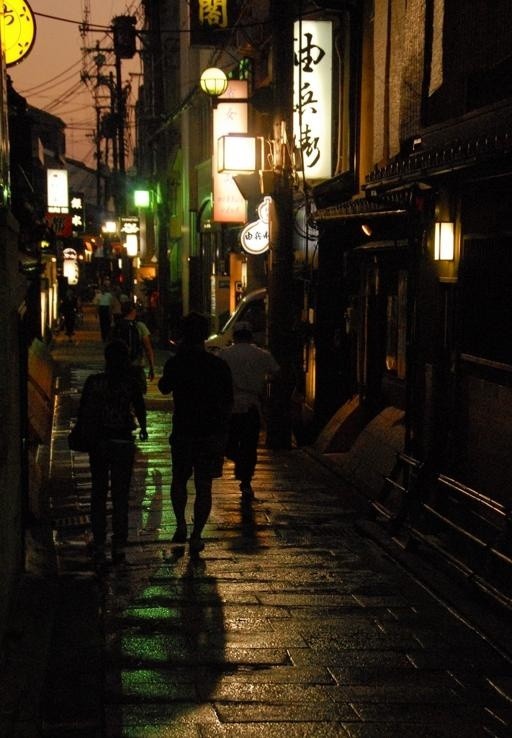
left=204, top=287, right=267, bottom=355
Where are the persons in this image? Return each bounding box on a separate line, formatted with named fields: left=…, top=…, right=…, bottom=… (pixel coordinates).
left=157, top=313, right=234, bottom=543
left=93, top=285, right=156, bottom=437
left=214, top=324, right=273, bottom=481
left=71, top=341, right=148, bottom=546
left=333, top=305, right=360, bottom=384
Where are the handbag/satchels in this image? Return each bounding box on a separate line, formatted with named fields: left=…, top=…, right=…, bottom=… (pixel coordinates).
left=70, top=422, right=87, bottom=452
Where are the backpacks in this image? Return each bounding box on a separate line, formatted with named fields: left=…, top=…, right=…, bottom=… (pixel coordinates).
left=113, top=318, right=139, bottom=356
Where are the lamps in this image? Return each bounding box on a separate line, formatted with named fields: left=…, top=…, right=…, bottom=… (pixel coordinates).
left=196, top=66, right=272, bottom=113
left=217, top=129, right=292, bottom=187
left=432, top=220, right=455, bottom=260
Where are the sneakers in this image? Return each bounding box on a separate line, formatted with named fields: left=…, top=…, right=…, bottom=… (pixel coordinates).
left=95, top=530, right=127, bottom=545
left=173, top=525, right=201, bottom=543
